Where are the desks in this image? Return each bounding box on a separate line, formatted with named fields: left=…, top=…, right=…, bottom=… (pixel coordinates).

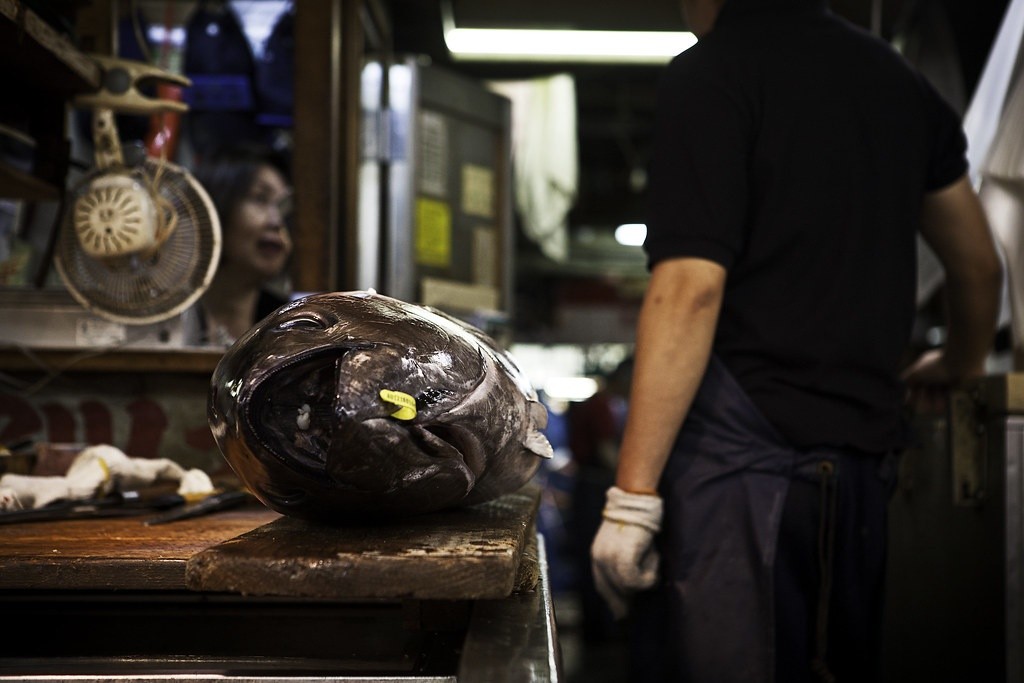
left=0, top=474, right=544, bottom=683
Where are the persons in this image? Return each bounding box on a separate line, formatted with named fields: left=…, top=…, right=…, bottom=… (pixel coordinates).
left=589, top=2, right=1005, bottom=683
left=174, top=141, right=306, bottom=352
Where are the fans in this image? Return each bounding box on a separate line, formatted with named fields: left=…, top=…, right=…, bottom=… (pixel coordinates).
left=54, top=55, right=223, bottom=326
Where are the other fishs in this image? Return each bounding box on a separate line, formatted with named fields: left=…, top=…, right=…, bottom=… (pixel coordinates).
left=210, top=290, right=555, bottom=523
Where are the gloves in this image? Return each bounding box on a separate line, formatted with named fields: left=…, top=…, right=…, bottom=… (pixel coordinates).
left=900, top=348, right=950, bottom=406
left=590, top=486, right=664, bottom=619
